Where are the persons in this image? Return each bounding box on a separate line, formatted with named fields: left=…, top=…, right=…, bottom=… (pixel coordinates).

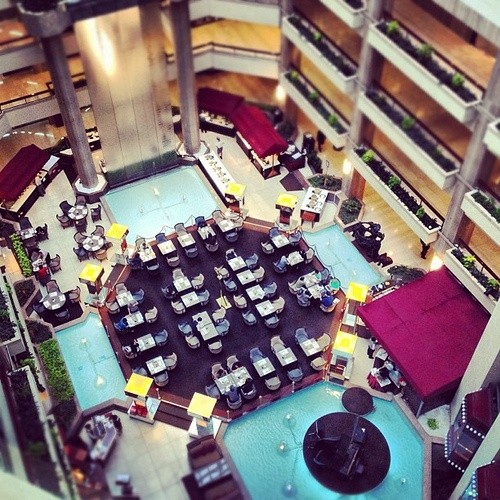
left=321, top=290, right=333, bottom=310
left=217, top=137, right=223, bottom=160
left=231, top=361, right=243, bottom=370
left=85, top=424, right=104, bottom=445
left=38, top=265, right=47, bottom=277
left=240, top=378, right=255, bottom=393
left=229, top=385, right=239, bottom=403
left=214, top=368, right=227, bottom=379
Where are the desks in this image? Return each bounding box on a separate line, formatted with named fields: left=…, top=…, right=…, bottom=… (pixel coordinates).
left=67, top=204, right=89, bottom=223
left=19, top=227, right=38, bottom=244
left=81, top=233, right=106, bottom=253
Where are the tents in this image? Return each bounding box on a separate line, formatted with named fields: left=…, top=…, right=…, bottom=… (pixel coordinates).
left=351, top=249, right=489, bottom=421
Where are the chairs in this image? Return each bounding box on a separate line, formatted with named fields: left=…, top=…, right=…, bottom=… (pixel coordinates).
left=25, top=242, right=83, bottom=322
left=60, top=201, right=73, bottom=213
left=36, top=223, right=50, bottom=240
left=54, top=214, right=75, bottom=229
left=106, top=209, right=340, bottom=413
left=94, top=247, right=108, bottom=263
left=90, top=204, right=102, bottom=222
left=74, top=219, right=89, bottom=233
left=91, top=226, right=105, bottom=238
left=72, top=231, right=89, bottom=244
left=19, top=217, right=33, bottom=230
left=72, top=247, right=91, bottom=262
left=73, top=193, right=87, bottom=207
